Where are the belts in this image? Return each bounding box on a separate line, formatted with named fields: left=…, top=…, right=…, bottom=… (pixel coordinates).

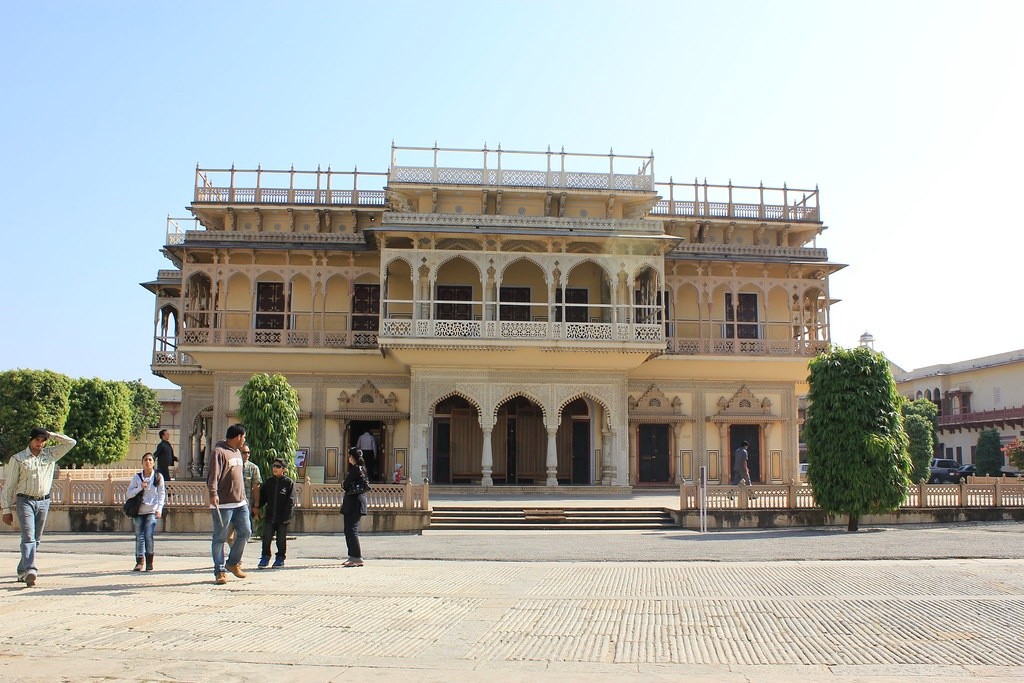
left=17, top=493, right=50, bottom=501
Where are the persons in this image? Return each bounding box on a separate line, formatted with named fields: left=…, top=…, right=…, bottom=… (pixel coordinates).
left=126, top=452, right=165, bottom=571
left=200, top=446, right=205, bottom=476
left=727, top=441, right=758, bottom=500
left=152, top=429, right=179, bottom=498
left=1, top=427, right=77, bottom=586
left=206, top=423, right=252, bottom=582
left=340, top=447, right=367, bottom=567
left=252, top=457, right=297, bottom=568
left=357, top=426, right=377, bottom=482
left=225, top=442, right=263, bottom=567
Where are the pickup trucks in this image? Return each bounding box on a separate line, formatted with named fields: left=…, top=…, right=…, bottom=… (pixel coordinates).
left=929, top=458, right=962, bottom=485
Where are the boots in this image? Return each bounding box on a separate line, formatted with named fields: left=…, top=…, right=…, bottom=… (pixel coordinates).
left=134, top=556, right=144, bottom=571
left=145, top=553, right=154, bottom=570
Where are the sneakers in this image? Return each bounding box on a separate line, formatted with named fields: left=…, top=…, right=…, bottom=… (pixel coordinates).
left=258, top=558, right=269, bottom=567
left=225, top=560, right=246, bottom=577
left=342, top=555, right=364, bottom=567
left=272, top=559, right=284, bottom=569
left=216, top=571, right=228, bottom=584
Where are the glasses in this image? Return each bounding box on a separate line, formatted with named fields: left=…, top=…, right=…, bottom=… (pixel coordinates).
left=240, top=450, right=250, bottom=455
left=273, top=464, right=284, bottom=469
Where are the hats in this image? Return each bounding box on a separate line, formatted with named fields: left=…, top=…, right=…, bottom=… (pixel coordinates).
left=395, top=463, right=403, bottom=471
left=29, top=427, right=48, bottom=442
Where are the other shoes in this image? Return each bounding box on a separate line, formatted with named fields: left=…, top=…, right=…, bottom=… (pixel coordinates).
left=727, top=495, right=734, bottom=500
left=749, top=496, right=758, bottom=499
left=26, top=569, right=37, bottom=586
left=18, top=574, right=26, bottom=582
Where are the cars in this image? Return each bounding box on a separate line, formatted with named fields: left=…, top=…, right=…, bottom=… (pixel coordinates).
left=946, top=459, right=1021, bottom=484
left=799, top=463, right=811, bottom=483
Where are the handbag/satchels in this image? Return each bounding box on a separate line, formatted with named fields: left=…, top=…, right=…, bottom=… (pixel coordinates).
left=348, top=467, right=371, bottom=495
left=121, top=473, right=144, bottom=519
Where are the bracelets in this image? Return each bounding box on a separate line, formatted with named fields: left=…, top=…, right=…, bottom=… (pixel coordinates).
left=253, top=504, right=259, bottom=509
